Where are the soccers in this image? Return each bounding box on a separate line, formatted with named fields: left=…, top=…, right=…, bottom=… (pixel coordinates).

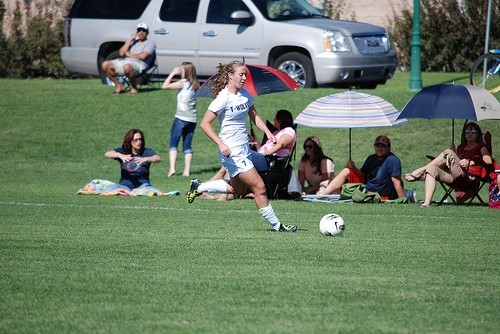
left=319, top=213, right=345, bottom=236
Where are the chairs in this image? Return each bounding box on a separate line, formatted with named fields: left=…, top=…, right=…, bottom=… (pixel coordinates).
left=242, top=120, right=297, bottom=197
left=426, top=132, right=495, bottom=206
left=119, top=47, right=160, bottom=92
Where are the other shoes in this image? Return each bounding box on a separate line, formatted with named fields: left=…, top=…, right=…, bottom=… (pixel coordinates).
left=166, top=190, right=180, bottom=196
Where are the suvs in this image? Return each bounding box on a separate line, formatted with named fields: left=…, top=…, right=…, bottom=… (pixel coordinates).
left=57, top=0, right=398, bottom=89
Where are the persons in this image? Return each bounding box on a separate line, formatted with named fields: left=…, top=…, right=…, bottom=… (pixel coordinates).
left=161, top=61, right=200, bottom=178
left=297, top=135, right=336, bottom=199
left=186, top=60, right=297, bottom=233
left=403, top=122, right=493, bottom=208
left=197, top=109, right=297, bottom=201
left=313, top=135, right=406, bottom=200
left=101, top=22, right=156, bottom=96
left=104, top=128, right=161, bottom=195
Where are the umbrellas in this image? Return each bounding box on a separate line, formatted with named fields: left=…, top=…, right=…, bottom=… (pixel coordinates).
left=292, top=91, right=409, bottom=161
left=190, top=62, right=302, bottom=138
left=395, top=83, right=500, bottom=152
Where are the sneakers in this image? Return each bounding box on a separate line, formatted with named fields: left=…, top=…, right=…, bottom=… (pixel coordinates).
left=187, top=178, right=203, bottom=203
left=275, top=222, right=297, bottom=232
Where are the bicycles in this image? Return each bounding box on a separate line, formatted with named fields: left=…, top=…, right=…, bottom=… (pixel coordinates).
left=469, top=49, right=500, bottom=94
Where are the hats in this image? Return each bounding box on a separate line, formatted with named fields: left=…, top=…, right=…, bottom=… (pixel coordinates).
left=137, top=24, right=149, bottom=32
left=374, top=136, right=391, bottom=148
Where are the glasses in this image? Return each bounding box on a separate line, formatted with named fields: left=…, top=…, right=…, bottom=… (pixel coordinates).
left=304, top=144, right=313, bottom=149
left=133, top=138, right=143, bottom=142
left=465, top=130, right=477, bottom=135
left=376, top=143, right=385, bottom=148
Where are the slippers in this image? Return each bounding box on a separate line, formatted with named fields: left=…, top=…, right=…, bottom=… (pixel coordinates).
left=404, top=174, right=425, bottom=182
